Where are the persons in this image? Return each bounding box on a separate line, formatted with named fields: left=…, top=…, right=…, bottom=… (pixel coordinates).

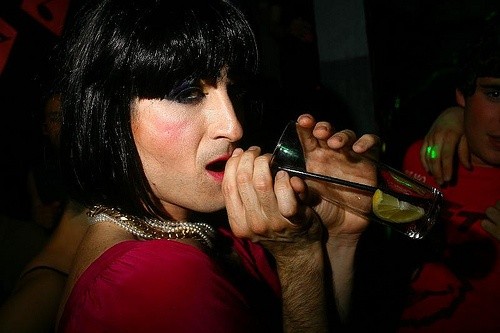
left=53, top=0, right=378, bottom=333
left=416, top=105, right=472, bottom=186
left=361, top=47, right=499, bottom=333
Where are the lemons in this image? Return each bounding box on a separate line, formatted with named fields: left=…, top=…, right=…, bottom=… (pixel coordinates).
left=372, top=189, right=425, bottom=223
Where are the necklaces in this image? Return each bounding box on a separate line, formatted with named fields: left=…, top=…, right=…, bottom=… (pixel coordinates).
left=85, top=200, right=221, bottom=257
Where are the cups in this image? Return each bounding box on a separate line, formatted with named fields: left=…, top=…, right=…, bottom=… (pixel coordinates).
left=265, top=120, right=445, bottom=244
